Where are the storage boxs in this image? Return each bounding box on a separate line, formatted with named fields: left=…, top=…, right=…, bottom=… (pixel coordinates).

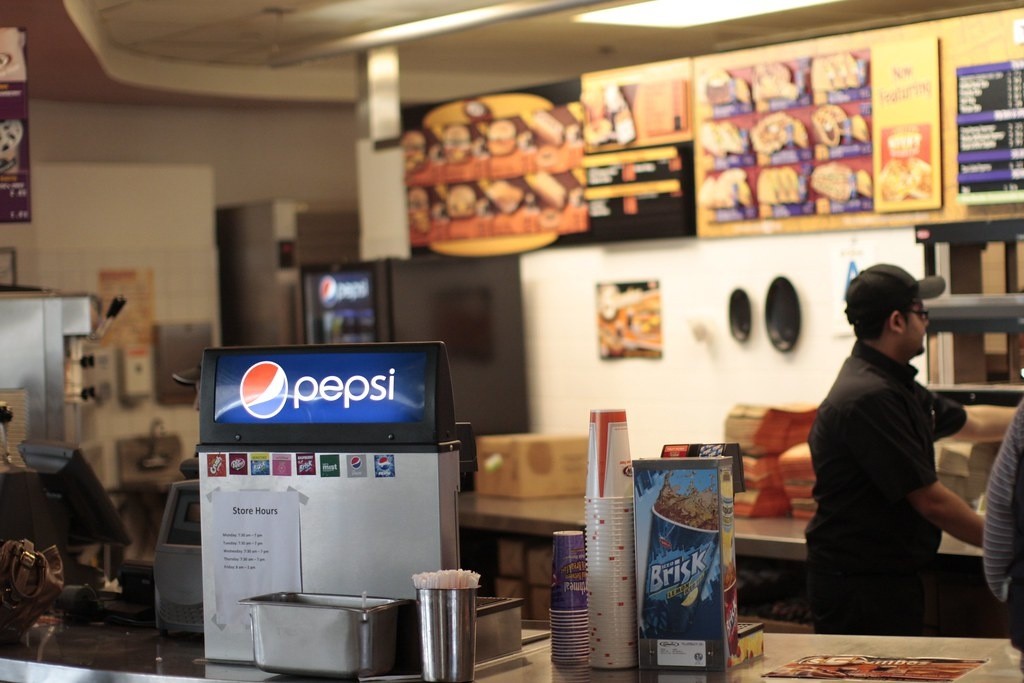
left=484, top=541, right=555, bottom=630
left=471, top=433, right=589, bottom=500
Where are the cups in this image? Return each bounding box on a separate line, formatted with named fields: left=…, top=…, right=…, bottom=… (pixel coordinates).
left=640, top=504, right=719, bottom=639
left=550, top=410, right=638, bottom=669
left=415, top=587, right=479, bottom=683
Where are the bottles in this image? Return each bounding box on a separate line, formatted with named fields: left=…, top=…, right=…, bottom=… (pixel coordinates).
left=325, top=310, right=374, bottom=343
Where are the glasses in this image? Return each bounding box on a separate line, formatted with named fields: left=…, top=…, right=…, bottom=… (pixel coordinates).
left=907, top=309, right=928, bottom=322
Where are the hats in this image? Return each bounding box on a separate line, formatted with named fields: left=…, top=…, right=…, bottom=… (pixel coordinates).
left=844, top=264, right=945, bottom=324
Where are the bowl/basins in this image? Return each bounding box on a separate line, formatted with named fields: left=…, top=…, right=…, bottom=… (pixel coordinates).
left=236, top=591, right=412, bottom=680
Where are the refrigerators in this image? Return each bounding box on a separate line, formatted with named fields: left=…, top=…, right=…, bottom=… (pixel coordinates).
left=301, top=255, right=529, bottom=433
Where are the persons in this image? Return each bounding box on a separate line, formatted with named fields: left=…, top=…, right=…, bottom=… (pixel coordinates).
left=804, top=264, right=1018, bottom=639
left=982, top=394, right=1024, bottom=668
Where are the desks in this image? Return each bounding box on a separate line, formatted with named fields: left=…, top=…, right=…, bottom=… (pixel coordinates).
left=457, top=482, right=1024, bottom=618
